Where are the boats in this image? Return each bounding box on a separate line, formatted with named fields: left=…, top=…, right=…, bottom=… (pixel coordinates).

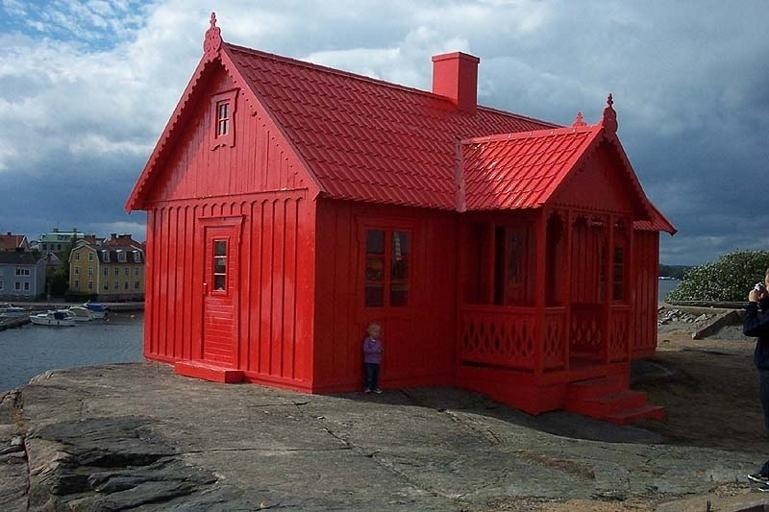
left=658, top=276, right=675, bottom=280
left=0, top=302, right=107, bottom=327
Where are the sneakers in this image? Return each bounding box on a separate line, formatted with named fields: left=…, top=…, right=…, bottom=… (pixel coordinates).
left=747, top=472, right=769, bottom=492
left=365, top=387, right=383, bottom=394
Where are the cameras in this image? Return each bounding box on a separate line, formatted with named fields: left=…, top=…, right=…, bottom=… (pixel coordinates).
left=755, top=281, right=766, bottom=293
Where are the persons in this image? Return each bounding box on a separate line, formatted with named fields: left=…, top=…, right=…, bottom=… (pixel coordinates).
left=743, top=268, right=769, bottom=492
left=362, top=323, right=384, bottom=394
